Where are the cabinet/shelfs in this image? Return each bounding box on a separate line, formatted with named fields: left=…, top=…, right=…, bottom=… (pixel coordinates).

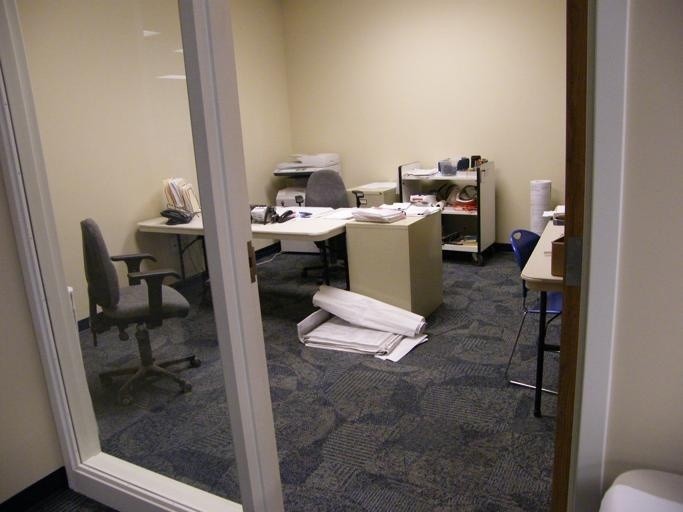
left=398, top=161, right=497, bottom=265
left=344, top=202, right=443, bottom=323
left=347, top=182, right=397, bottom=205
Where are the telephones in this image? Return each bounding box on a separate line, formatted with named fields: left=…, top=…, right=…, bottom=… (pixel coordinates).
left=160, top=208, right=192, bottom=225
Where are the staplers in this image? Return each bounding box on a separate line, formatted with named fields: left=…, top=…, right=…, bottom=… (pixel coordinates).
left=276, top=209, right=296, bottom=224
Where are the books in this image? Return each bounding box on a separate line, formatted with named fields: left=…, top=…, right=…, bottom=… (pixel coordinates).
left=462, top=234, right=478, bottom=247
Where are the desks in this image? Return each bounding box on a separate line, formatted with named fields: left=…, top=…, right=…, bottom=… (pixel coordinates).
left=521, top=209, right=564, bottom=419
left=137, top=205, right=357, bottom=298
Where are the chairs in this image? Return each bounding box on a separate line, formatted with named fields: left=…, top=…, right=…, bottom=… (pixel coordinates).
left=504, top=229, right=563, bottom=394
left=295, top=169, right=348, bottom=285
left=79, top=218, right=201, bottom=405
